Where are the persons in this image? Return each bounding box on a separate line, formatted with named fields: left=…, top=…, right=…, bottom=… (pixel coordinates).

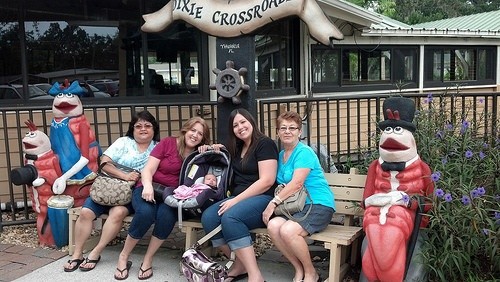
left=201, top=108, right=279, bottom=282
left=181, top=174, right=217, bottom=199
left=64, top=110, right=162, bottom=272
left=263, top=112, right=336, bottom=282
left=115, top=117, right=211, bottom=280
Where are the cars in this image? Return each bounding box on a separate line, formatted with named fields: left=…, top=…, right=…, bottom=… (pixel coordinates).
left=0, top=84, right=55, bottom=99
left=80, top=82, right=110, bottom=98
left=87, top=80, right=119, bottom=96
left=35, top=83, right=52, bottom=93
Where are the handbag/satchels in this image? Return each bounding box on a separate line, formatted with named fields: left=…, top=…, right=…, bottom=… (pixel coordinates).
left=180, top=247, right=224, bottom=282
left=274, top=188, right=307, bottom=215
left=89, top=162, right=141, bottom=205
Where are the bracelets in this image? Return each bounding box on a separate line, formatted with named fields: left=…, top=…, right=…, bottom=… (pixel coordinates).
left=270, top=200, right=278, bottom=207
left=275, top=195, right=282, bottom=203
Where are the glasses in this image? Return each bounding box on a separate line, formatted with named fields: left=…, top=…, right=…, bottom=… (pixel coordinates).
left=279, top=127, right=300, bottom=131
left=134, top=123, right=154, bottom=130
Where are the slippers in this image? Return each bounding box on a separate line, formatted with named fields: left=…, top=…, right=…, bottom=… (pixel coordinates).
left=115, top=261, right=132, bottom=280
left=138, top=263, right=153, bottom=279
left=79, top=255, right=100, bottom=271
left=223, top=272, right=248, bottom=282
left=64, top=258, right=85, bottom=272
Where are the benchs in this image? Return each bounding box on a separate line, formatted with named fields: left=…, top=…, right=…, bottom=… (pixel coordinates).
left=64, top=167, right=367, bottom=281
